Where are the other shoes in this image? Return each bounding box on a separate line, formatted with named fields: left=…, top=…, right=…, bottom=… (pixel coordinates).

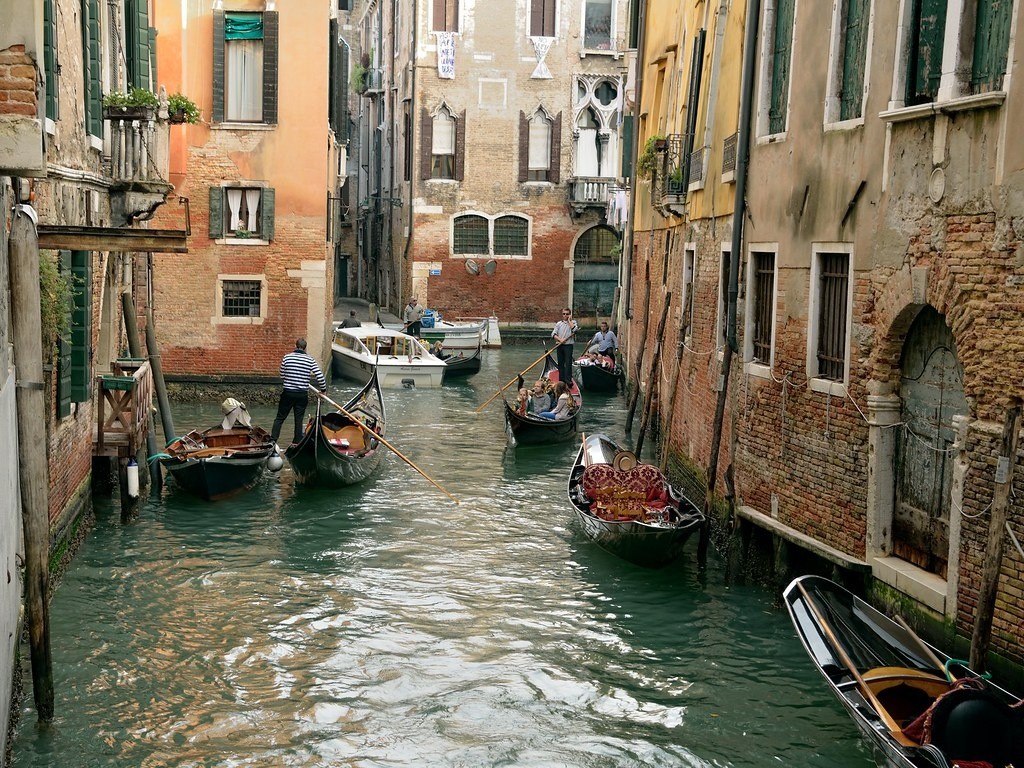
left=291, top=440, right=298, bottom=446
left=266, top=439, right=277, bottom=447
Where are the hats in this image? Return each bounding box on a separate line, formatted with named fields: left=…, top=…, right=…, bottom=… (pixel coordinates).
left=613, top=451, right=637, bottom=472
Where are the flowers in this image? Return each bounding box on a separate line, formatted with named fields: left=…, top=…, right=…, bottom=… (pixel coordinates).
left=235, top=220, right=251, bottom=238
left=167, top=93, right=204, bottom=125
left=102, top=86, right=160, bottom=111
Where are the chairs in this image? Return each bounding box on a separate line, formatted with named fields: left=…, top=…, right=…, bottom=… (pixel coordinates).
left=596, top=484, right=627, bottom=515
left=614, top=487, right=647, bottom=520
left=918, top=690, right=1024, bottom=768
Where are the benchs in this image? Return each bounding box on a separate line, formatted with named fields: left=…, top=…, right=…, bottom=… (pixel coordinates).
left=579, top=356, right=614, bottom=371
left=250, top=427, right=270, bottom=444
left=306, top=413, right=365, bottom=449
left=224, top=444, right=269, bottom=448
left=584, top=464, right=679, bottom=522
left=168, top=432, right=204, bottom=455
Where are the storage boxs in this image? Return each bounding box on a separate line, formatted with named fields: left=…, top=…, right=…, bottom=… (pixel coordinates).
left=102, top=375, right=135, bottom=390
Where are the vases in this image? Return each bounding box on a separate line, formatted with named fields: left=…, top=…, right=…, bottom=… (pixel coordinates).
left=170, top=108, right=185, bottom=123
left=236, top=231, right=248, bottom=238
left=108, top=106, right=154, bottom=119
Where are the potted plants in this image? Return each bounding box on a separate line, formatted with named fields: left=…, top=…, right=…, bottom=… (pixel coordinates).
left=636, top=133, right=684, bottom=194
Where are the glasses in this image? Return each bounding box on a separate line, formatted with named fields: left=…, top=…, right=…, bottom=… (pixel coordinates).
left=413, top=300, right=417, bottom=302
left=563, top=313, right=569, bottom=315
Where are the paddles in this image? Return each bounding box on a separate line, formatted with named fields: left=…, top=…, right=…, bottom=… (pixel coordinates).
left=797, top=581, right=901, bottom=732
left=581, top=339, right=592, bottom=357
left=581, top=431, right=588, bottom=468
left=895, top=614, right=964, bottom=689
left=309, top=384, right=460, bottom=504
left=475, top=327, right=582, bottom=411
left=398, top=318, right=417, bottom=332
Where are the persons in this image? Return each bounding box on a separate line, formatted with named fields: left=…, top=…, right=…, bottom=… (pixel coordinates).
left=586, top=322, right=620, bottom=364
left=529, top=380, right=551, bottom=414
left=337, top=310, right=362, bottom=329
left=404, top=297, right=425, bottom=338
left=586, top=352, right=609, bottom=370
left=271, top=338, right=327, bottom=445
left=539, top=380, right=575, bottom=420
left=515, top=388, right=528, bottom=416
left=429, top=341, right=445, bottom=360
left=549, top=307, right=579, bottom=388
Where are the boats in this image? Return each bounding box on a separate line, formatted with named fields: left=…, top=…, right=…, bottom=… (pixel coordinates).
left=572, top=353, right=623, bottom=393
left=331, top=310, right=502, bottom=350
left=158, top=420, right=275, bottom=501
left=499, top=341, right=581, bottom=449
left=284, top=368, right=387, bottom=487
left=429, top=338, right=483, bottom=381
left=782, top=574, right=1024, bottom=768
left=332, top=327, right=448, bottom=391
left=566, top=433, right=705, bottom=571
